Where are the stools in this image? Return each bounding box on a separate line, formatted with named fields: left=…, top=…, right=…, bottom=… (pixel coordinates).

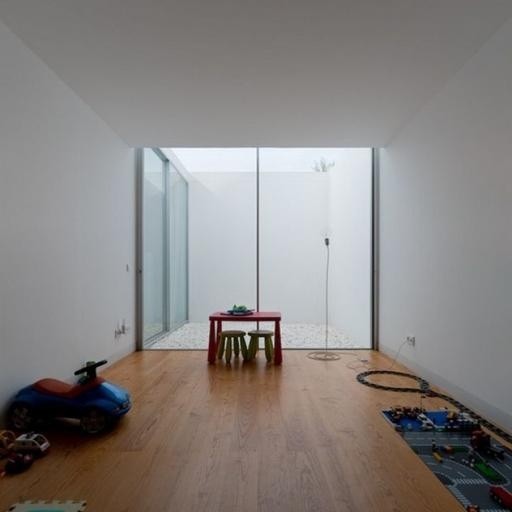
left=218, top=330, right=248, bottom=364
left=247, top=329, right=274, bottom=362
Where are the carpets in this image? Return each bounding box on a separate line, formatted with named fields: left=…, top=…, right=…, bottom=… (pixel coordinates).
left=379, top=410, right=512, bottom=511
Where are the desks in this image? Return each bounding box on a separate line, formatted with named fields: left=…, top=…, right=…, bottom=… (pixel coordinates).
left=207, top=310, right=283, bottom=366
